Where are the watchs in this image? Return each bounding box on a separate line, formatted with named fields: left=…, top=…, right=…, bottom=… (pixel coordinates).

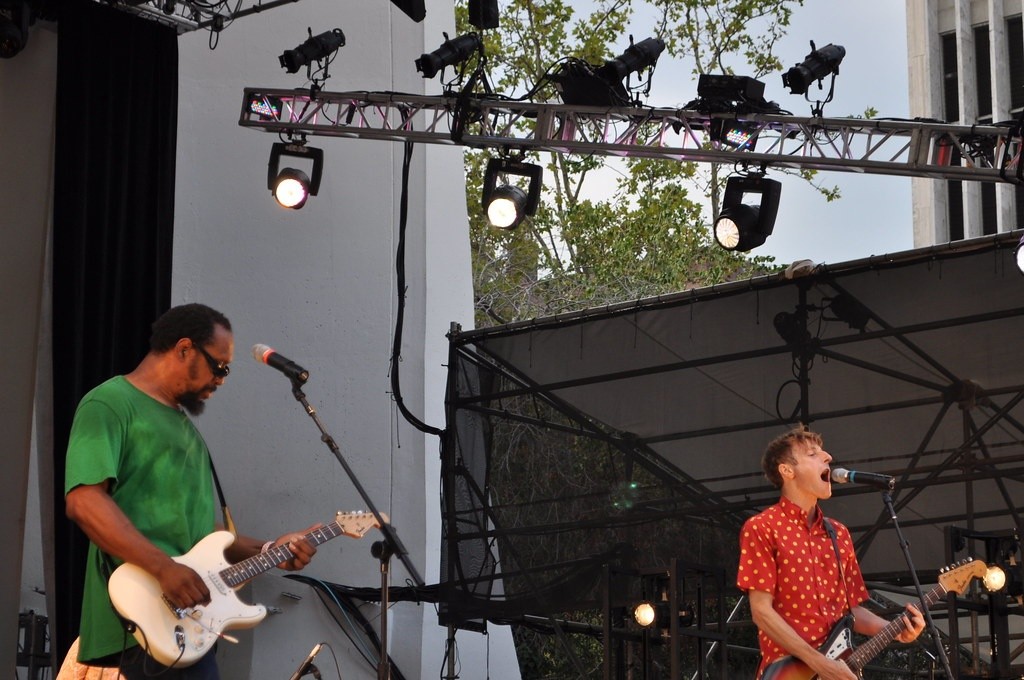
left=261, top=539, right=275, bottom=555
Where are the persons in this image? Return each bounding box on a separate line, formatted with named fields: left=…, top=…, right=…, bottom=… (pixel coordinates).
left=736, top=426, right=926, bottom=679
left=63, top=303, right=318, bottom=680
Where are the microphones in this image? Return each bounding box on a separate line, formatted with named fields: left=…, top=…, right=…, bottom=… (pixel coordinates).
left=831, top=468, right=895, bottom=486
left=251, top=343, right=309, bottom=383
left=289, top=643, right=323, bottom=680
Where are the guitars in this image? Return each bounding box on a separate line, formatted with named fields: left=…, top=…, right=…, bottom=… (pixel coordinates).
left=762, top=555, right=988, bottom=679
left=106, top=509, right=390, bottom=672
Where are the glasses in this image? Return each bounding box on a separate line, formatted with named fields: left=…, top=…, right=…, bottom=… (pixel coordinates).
left=187, top=337, right=230, bottom=377
